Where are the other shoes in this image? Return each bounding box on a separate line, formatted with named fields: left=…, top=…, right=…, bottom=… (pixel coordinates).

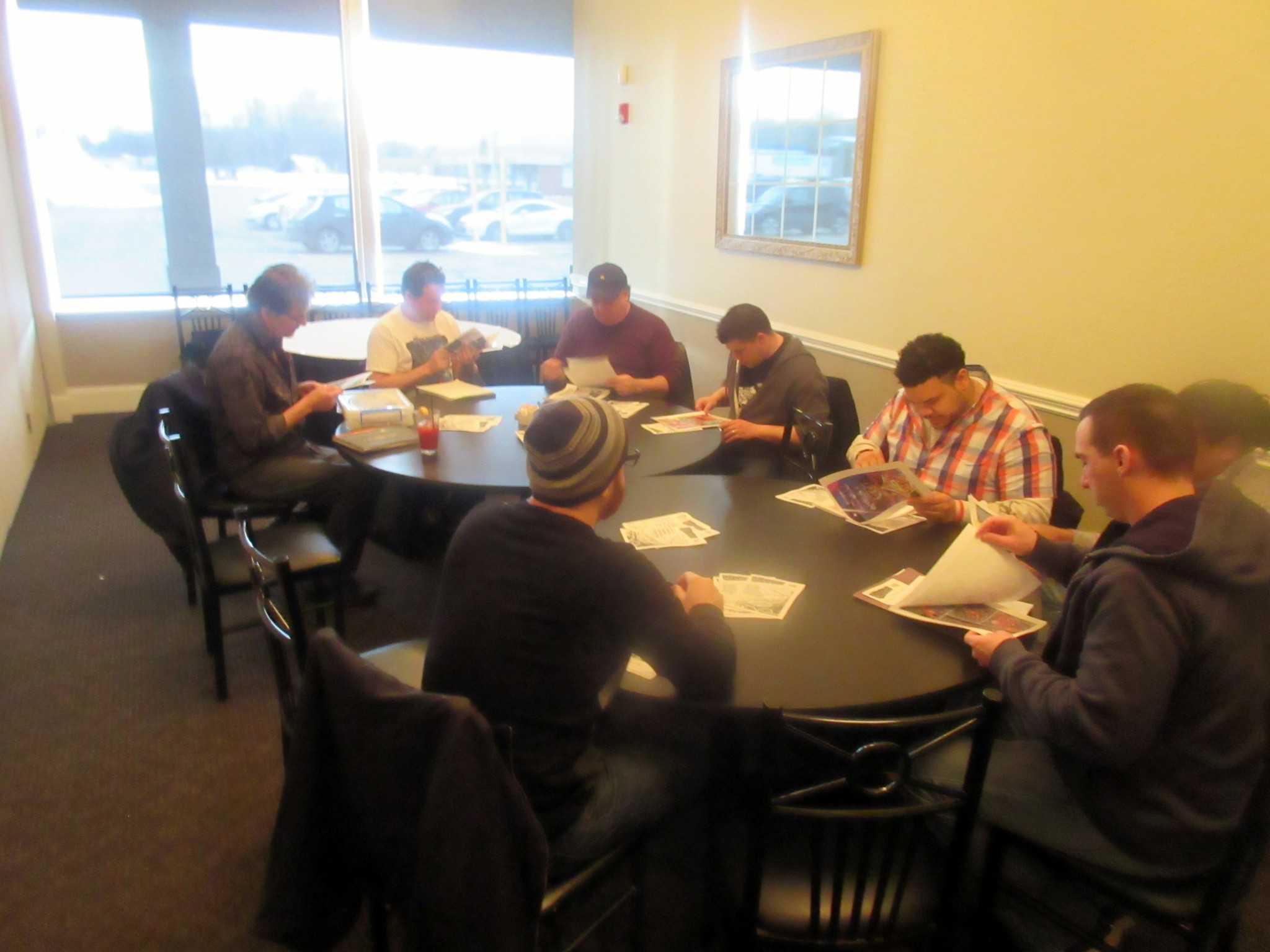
left=345, top=580, right=378, bottom=605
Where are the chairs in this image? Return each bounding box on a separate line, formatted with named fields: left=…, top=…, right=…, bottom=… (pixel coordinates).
left=154, top=268, right=1270, bottom=951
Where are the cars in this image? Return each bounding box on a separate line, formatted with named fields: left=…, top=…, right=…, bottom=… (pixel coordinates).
left=458, top=198, right=576, bottom=243
left=406, top=184, right=481, bottom=213
left=247, top=189, right=455, bottom=253
left=430, top=190, right=540, bottom=229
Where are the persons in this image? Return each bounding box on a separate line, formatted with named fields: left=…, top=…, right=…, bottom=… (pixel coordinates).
left=846, top=335, right=1057, bottom=527
left=368, top=261, right=481, bottom=389
left=695, top=304, right=833, bottom=477
left=205, top=265, right=378, bottom=606
left=911, top=377, right=1270, bottom=952
left=537, top=261, right=680, bottom=402
left=422, top=393, right=734, bottom=951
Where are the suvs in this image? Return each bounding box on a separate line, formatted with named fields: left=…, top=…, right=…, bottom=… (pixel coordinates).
left=743, top=182, right=850, bottom=238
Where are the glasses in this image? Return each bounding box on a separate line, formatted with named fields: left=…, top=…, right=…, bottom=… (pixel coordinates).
left=620, top=447, right=640, bottom=467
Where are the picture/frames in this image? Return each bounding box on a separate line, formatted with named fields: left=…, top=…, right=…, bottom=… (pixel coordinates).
left=715, top=30, right=880, bottom=265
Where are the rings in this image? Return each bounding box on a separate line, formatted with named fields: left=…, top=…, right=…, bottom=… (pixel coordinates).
left=732, top=430, right=735, bottom=434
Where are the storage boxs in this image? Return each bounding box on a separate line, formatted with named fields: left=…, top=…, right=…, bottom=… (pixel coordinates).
left=336, top=387, right=415, bottom=430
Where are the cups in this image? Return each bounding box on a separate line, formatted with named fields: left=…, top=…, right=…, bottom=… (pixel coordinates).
left=412, top=408, right=441, bottom=455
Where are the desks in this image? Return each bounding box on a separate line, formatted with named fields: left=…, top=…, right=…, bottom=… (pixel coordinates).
left=585, top=471, right=1045, bottom=726
left=336, top=384, right=723, bottom=492
left=282, top=317, right=521, bottom=430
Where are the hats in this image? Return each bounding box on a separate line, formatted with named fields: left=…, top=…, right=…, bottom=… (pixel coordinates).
left=524, top=396, right=629, bottom=505
left=587, top=263, right=627, bottom=298
